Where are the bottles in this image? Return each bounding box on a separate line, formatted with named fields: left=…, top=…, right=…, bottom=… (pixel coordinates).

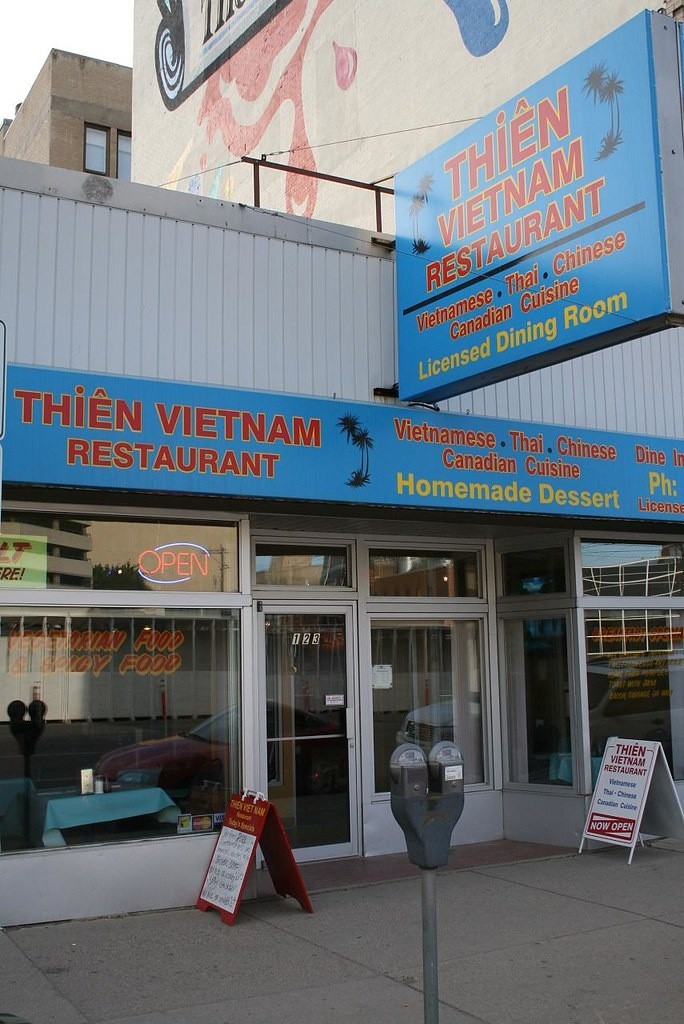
left=104, top=773, right=111, bottom=794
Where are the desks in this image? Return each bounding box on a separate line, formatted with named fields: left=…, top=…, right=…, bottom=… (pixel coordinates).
left=25, top=782, right=182, bottom=849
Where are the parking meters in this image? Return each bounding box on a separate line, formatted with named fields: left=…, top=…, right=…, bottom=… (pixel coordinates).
left=390, top=741, right=465, bottom=1024
left=8, top=700, right=47, bottom=850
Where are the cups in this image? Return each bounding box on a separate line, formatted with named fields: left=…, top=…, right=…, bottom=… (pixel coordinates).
left=94, top=774, right=104, bottom=795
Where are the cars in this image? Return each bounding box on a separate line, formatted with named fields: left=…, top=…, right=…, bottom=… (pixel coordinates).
left=95, top=700, right=347, bottom=814
left=392, top=702, right=486, bottom=787
left=585, top=654, right=684, bottom=778
